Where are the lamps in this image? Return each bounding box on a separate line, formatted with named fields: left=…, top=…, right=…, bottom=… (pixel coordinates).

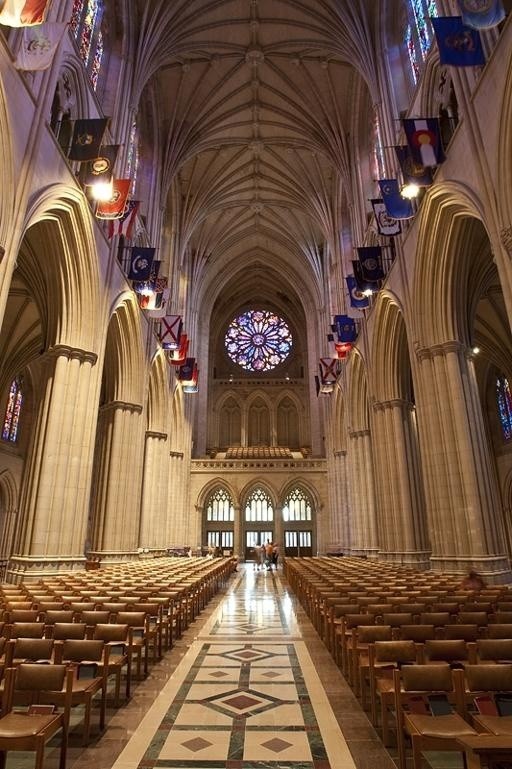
left=399, top=184, right=430, bottom=199
left=93, top=172, right=115, bottom=201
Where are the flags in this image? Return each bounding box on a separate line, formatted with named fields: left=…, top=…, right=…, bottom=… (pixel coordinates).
left=15, top=22, right=67, bottom=71
left=315, top=118, right=447, bottom=397
left=0, top=0, right=50, bottom=27
left=68, top=118, right=199, bottom=393
left=431, top=17, right=485, bottom=66
left=459, top=0, right=505, bottom=30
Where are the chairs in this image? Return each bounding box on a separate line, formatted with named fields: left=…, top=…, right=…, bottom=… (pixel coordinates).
left=341, top=614, right=379, bottom=685
left=112, top=612, right=150, bottom=679
left=352, top=625, right=395, bottom=706
left=77, top=624, right=133, bottom=707
left=77, top=612, right=112, bottom=624
left=3, top=638, right=57, bottom=685
left=68, top=602, right=97, bottom=612
left=130, top=603, right=163, bottom=666
left=388, top=664, right=480, bottom=769
left=5, top=601, right=34, bottom=610
left=421, top=639, right=474, bottom=664
left=98, top=602, right=127, bottom=612
left=462, top=602, right=493, bottom=612
left=50, top=622, right=89, bottom=640
left=281, top=556, right=512, bottom=604
left=492, top=610, right=512, bottom=623
left=117, top=596, right=144, bottom=603
left=1, top=555, right=235, bottom=596
left=224, top=447, right=294, bottom=460
left=474, top=639, right=512, bottom=665
left=6, top=610, right=42, bottom=622
left=494, top=601, right=512, bottom=611
left=145, top=597, right=174, bottom=651
left=395, top=625, right=439, bottom=641
left=9, top=622, right=49, bottom=638
left=51, top=639, right=112, bottom=741
left=417, top=612, right=453, bottom=625
left=439, top=625, right=484, bottom=639
left=397, top=604, right=429, bottom=613
left=86, top=595, right=115, bottom=602
left=458, top=664, right=512, bottom=769
left=329, top=605, right=363, bottom=666
left=30, top=595, right=57, bottom=602
left=379, top=612, right=417, bottom=625
left=3, top=596, right=29, bottom=601
left=58, top=595, right=85, bottom=602
left=35, top=602, right=66, bottom=610
left=453, top=611, right=491, bottom=624
left=368, top=641, right=421, bottom=747
left=42, top=609, right=77, bottom=623
left=484, top=623, right=512, bottom=639
left=364, top=605, right=396, bottom=613
left=430, top=603, right=462, bottom=613
left=1, top=662, right=76, bottom=769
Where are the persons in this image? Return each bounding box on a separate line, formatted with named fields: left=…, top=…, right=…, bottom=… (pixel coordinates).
left=188, top=548, right=239, bottom=573
left=254, top=542, right=280, bottom=571
left=462, top=570, right=487, bottom=590
